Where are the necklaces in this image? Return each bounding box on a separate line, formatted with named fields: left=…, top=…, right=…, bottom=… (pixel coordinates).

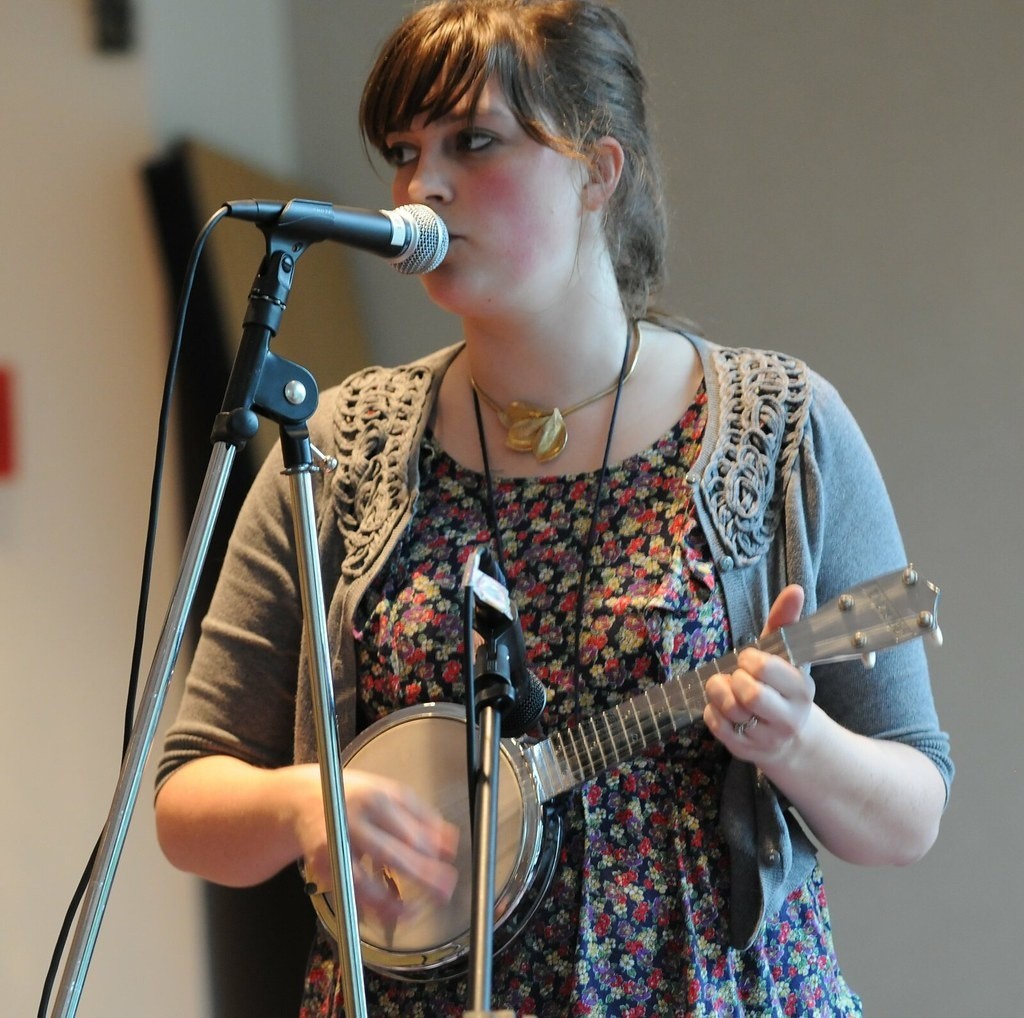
left=473, top=328, right=633, bottom=811
left=468, top=315, right=641, bottom=464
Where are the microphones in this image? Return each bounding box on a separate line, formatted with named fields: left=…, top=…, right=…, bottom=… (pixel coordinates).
left=474, top=546, right=546, bottom=740
left=222, top=199, right=449, bottom=275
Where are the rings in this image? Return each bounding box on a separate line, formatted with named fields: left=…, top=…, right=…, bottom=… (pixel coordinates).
left=733, top=717, right=758, bottom=736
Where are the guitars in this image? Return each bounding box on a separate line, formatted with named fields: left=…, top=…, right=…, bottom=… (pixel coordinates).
left=304, top=562, right=939, bottom=981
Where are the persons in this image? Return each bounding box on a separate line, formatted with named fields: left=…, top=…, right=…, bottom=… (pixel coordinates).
left=155, top=1, right=954, bottom=1018
